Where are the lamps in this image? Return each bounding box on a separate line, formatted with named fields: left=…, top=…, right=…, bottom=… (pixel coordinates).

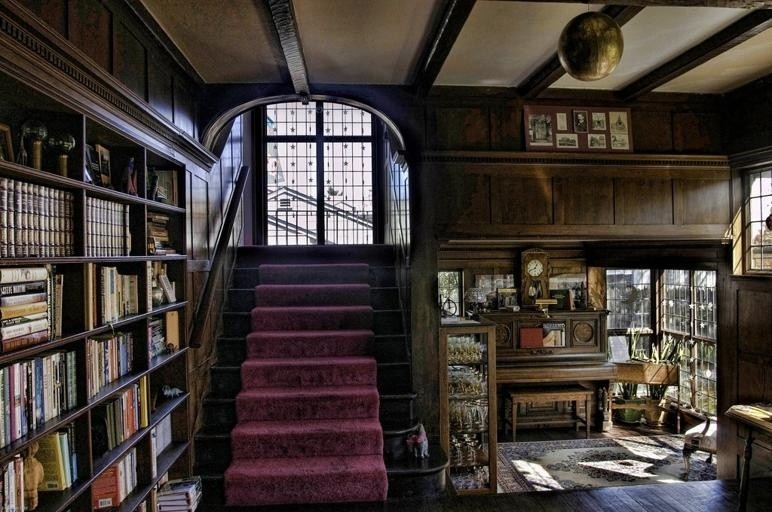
left=465, top=288, right=487, bottom=310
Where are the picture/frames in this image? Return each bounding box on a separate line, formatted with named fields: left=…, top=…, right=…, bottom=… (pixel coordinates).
left=522, top=105, right=632, bottom=154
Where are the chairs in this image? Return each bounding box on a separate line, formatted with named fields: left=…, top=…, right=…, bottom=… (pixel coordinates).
left=680, top=409, right=716, bottom=480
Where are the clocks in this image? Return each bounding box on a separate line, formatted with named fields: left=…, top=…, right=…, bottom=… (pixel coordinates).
left=527, top=259, right=544, bottom=277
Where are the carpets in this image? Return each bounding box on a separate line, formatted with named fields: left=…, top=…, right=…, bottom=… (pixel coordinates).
left=223, top=245, right=388, bottom=506
left=497, top=433, right=718, bottom=493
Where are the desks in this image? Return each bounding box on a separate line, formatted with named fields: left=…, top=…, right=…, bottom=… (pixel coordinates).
left=607, top=359, right=681, bottom=429
left=724, top=398, right=771, bottom=512
left=503, top=383, right=593, bottom=441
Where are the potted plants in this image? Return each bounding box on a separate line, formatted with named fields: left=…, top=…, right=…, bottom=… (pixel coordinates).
left=641, top=337, right=684, bottom=425
left=614, top=329, right=646, bottom=421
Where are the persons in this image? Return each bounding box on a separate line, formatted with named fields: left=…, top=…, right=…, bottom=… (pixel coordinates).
left=576, top=113, right=586, bottom=130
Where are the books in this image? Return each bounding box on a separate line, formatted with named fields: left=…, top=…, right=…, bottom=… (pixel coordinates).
left=146, top=168, right=179, bottom=206
left=87, top=196, right=132, bottom=256
left=2, top=329, right=48, bottom=351
left=0, top=291, right=48, bottom=307
left=0, top=301, right=48, bottom=320
left=0, top=351, right=79, bottom=450
left=0, top=281, right=48, bottom=296
left=91, top=375, right=149, bottom=451
left=91, top=447, right=137, bottom=509
left=36, top=422, right=79, bottom=492
left=52, top=265, right=56, bottom=340
left=0, top=318, right=48, bottom=341
left=0, top=312, right=48, bottom=328
left=55, top=274, right=63, bottom=337
left=86, top=332, right=134, bottom=399
left=0, top=454, right=27, bottom=512
left=147, top=212, right=178, bottom=255
left=155, top=413, right=172, bottom=457
left=151, top=317, right=167, bottom=359
left=43, top=264, right=52, bottom=342
left=152, top=261, right=177, bottom=308
left=0, top=266, right=49, bottom=285
left=0, top=178, right=83, bottom=258
left=156, top=474, right=204, bottom=511
left=94, top=263, right=138, bottom=328
left=150, top=426, right=158, bottom=479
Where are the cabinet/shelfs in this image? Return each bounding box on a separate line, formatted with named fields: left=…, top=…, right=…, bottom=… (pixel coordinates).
left=465, top=308, right=611, bottom=432
left=1, top=80, right=206, bottom=511
left=21, top=0, right=206, bottom=142
left=439, top=316, right=496, bottom=494
